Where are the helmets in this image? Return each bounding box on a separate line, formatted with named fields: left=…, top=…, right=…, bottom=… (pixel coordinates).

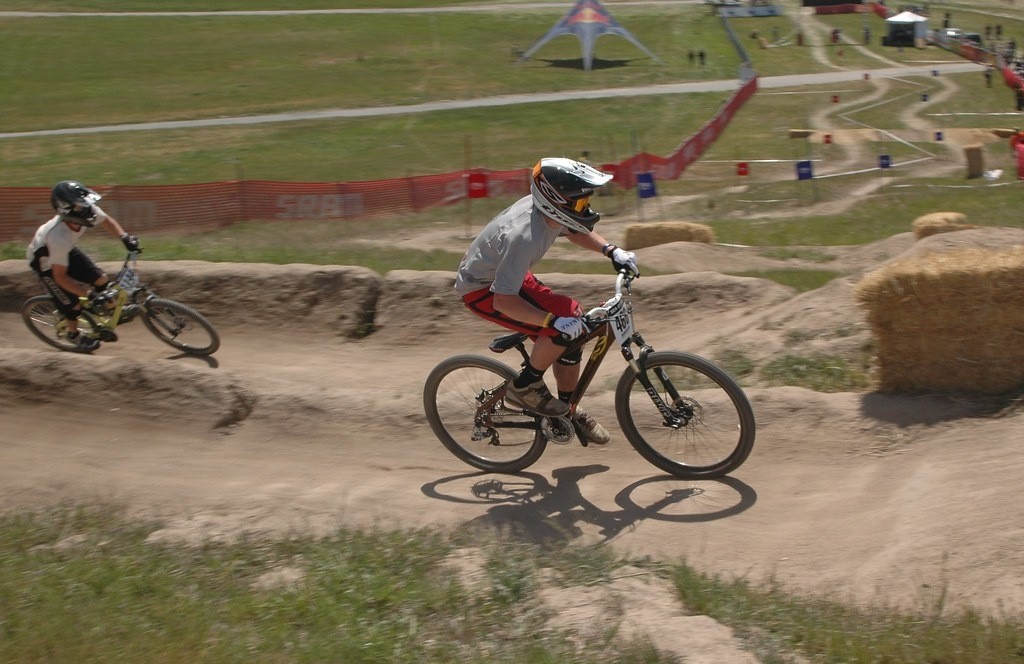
left=51, top=180, right=101, bottom=226
left=530, top=157, right=613, bottom=234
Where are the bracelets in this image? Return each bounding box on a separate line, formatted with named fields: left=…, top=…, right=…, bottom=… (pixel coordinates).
left=602, top=243, right=614, bottom=256
left=543, top=312, right=553, bottom=328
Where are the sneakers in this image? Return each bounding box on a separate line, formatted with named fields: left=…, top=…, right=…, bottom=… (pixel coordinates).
left=503, top=376, right=571, bottom=418
left=570, top=407, right=610, bottom=444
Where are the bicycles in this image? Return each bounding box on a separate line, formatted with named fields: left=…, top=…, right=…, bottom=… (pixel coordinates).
left=423, top=267, right=758, bottom=482
left=19, top=248, right=221, bottom=357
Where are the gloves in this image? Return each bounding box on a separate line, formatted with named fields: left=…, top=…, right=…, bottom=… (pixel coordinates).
left=121, top=234, right=142, bottom=252
left=86, top=290, right=106, bottom=307
left=543, top=313, right=584, bottom=341
left=603, top=244, right=640, bottom=279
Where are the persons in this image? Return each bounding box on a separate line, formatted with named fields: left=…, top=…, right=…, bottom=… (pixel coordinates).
left=26, top=180, right=142, bottom=351
left=454, top=157, right=639, bottom=445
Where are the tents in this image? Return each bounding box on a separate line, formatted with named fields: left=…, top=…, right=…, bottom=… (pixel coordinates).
left=884, top=10, right=929, bottom=47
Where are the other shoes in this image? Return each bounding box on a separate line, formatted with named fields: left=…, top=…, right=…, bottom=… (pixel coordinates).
left=66, top=330, right=100, bottom=349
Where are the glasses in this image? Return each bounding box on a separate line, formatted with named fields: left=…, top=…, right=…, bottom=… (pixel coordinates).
left=571, top=191, right=594, bottom=214
